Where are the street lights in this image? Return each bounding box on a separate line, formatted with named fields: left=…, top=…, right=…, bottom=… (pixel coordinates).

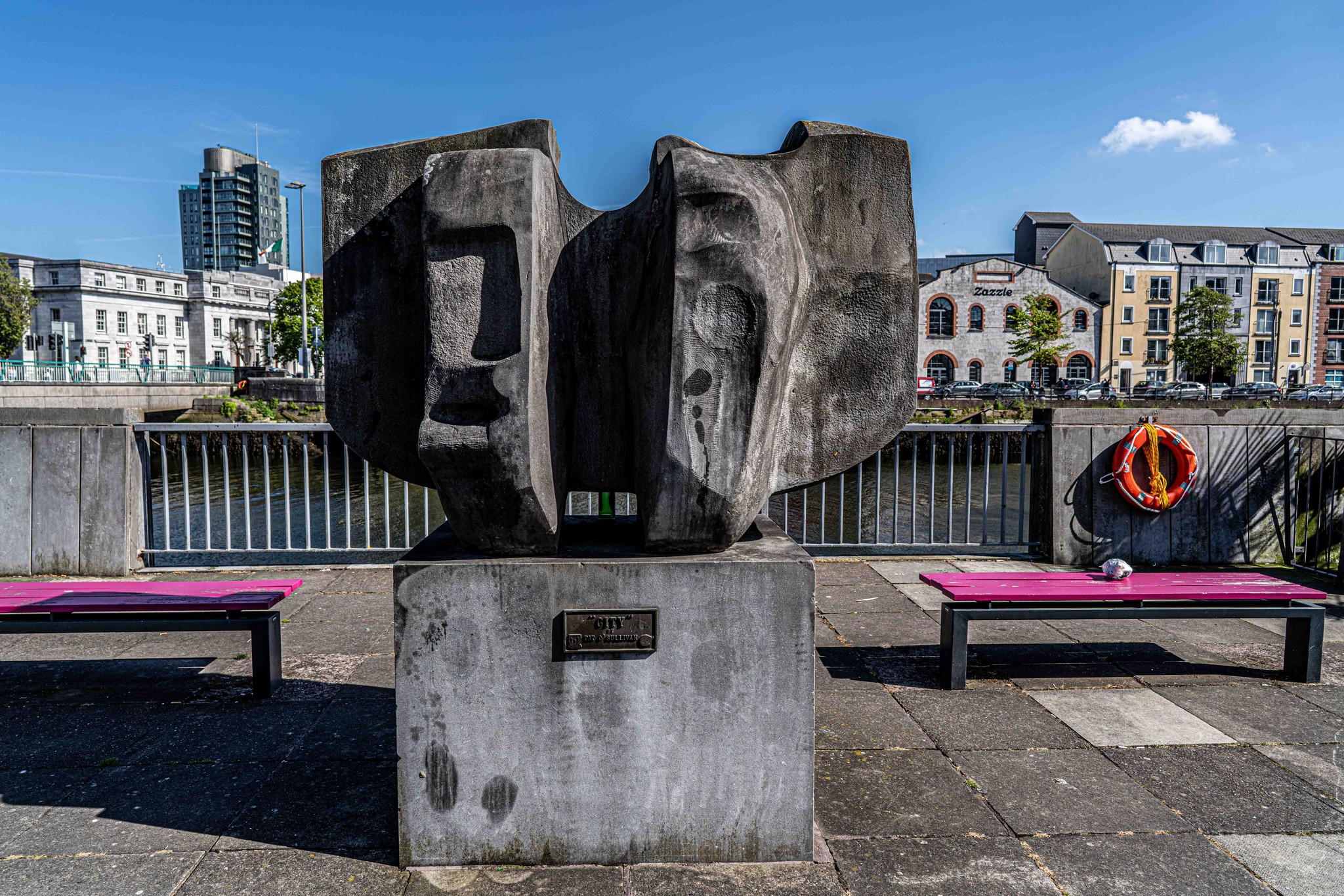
left=267, top=297, right=288, bottom=367
left=284, top=181, right=308, bottom=378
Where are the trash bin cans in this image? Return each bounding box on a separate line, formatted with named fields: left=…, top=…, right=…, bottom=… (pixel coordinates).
left=311, top=376, right=315, bottom=378
left=297, top=373, right=303, bottom=378
left=161, top=371, right=166, bottom=381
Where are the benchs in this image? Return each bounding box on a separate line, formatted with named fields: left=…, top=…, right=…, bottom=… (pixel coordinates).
left=921, top=571, right=1326, bottom=691
left=0, top=577, right=303, bottom=697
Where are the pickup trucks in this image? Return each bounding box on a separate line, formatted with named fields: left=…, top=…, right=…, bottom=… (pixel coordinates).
left=1016, top=380, right=1045, bottom=398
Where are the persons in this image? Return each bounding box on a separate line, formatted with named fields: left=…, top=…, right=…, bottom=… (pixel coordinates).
left=1029, top=381, right=1038, bottom=399
left=1098, top=378, right=1107, bottom=399
left=142, top=353, right=152, bottom=382
left=1058, top=377, right=1065, bottom=399
left=1104, top=379, right=1114, bottom=399
left=74, top=355, right=82, bottom=381
left=1174, top=377, right=1179, bottom=382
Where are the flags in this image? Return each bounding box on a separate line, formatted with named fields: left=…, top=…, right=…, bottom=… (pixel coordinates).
left=256, top=237, right=283, bottom=256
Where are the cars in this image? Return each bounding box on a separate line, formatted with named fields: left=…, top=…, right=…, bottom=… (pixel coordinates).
left=937, top=380, right=1030, bottom=400
left=1064, top=381, right=1117, bottom=400
left=269, top=368, right=292, bottom=379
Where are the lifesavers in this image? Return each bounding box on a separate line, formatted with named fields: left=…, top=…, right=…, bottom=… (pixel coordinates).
left=1113, top=424, right=1197, bottom=510
left=238, top=380, right=247, bottom=390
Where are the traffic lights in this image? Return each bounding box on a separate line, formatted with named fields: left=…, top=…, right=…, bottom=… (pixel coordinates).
left=25, top=335, right=33, bottom=351
left=47, top=334, right=55, bottom=350
left=57, top=334, right=63, bottom=345
left=80, top=347, right=86, bottom=356
left=142, top=335, right=151, bottom=351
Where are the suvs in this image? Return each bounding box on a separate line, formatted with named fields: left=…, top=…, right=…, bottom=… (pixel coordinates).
left=1053, top=377, right=1097, bottom=395
left=1285, top=383, right=1344, bottom=401
left=1132, top=380, right=1282, bottom=402
left=916, top=377, right=936, bottom=400
